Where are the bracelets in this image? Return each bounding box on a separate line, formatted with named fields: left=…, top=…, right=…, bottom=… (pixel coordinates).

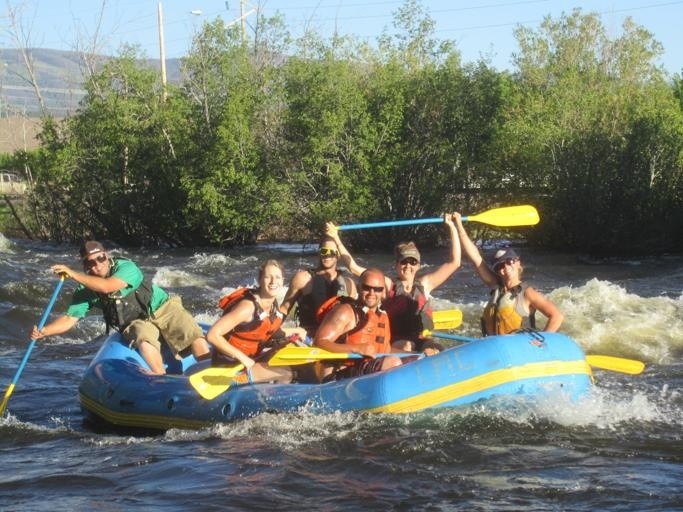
left=230, top=350, right=241, bottom=359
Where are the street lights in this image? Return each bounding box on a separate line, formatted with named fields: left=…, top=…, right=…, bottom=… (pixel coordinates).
left=189, top=9, right=202, bottom=18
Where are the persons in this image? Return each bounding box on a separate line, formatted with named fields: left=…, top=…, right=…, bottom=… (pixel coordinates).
left=313, top=267, right=419, bottom=383
left=29, top=238, right=208, bottom=374
left=205, top=258, right=306, bottom=387
left=276, top=236, right=358, bottom=338
left=453, top=211, right=564, bottom=337
left=324, top=213, right=462, bottom=360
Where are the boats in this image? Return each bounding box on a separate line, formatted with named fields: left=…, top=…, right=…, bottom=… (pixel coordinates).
left=72, top=320, right=603, bottom=442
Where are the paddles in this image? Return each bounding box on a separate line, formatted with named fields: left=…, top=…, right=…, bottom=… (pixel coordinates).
left=332, top=206, right=539, bottom=229
left=429, top=310, right=462, bottom=330
left=188, top=337, right=298, bottom=400
left=423, top=330, right=645, bottom=375
left=268, top=347, right=425, bottom=365
left=1, top=273, right=65, bottom=416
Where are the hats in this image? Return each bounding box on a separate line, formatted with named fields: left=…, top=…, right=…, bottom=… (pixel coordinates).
left=397, top=249, right=420, bottom=261
left=491, top=247, right=516, bottom=272
left=80, top=240, right=105, bottom=259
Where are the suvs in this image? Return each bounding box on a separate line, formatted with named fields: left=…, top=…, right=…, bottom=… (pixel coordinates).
left=0, top=168, right=31, bottom=196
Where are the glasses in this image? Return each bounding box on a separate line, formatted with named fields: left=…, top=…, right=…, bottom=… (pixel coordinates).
left=87, top=254, right=106, bottom=267
left=318, top=248, right=341, bottom=258
left=497, top=258, right=519, bottom=270
left=361, top=283, right=385, bottom=292
left=400, top=259, right=418, bottom=264
